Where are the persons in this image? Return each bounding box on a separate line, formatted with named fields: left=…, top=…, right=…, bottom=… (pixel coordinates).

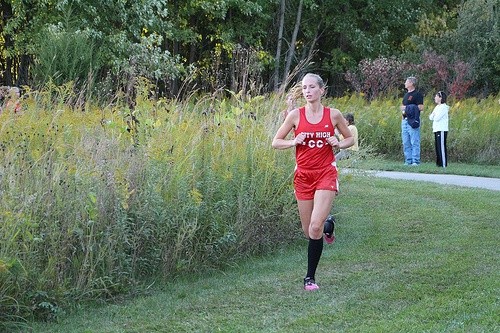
left=400, top=77, right=425, bottom=165
left=428, top=91, right=449, bottom=167
left=271, top=73, right=357, bottom=290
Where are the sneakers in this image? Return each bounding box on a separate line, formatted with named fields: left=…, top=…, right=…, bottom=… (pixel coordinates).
left=323, top=214, right=336, bottom=244
left=304, top=276, right=319, bottom=291
